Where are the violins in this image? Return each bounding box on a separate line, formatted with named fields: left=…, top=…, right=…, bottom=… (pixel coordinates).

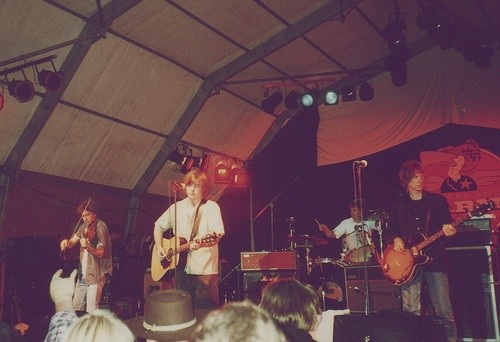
left=82, top=220, right=97, bottom=252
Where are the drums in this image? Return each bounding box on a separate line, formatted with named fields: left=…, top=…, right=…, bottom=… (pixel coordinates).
left=307, top=257, right=352, bottom=312
left=342, top=229, right=375, bottom=262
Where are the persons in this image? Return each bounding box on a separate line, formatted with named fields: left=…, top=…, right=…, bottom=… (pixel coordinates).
left=318, top=199, right=375, bottom=264
left=60, top=202, right=122, bottom=313
left=139, top=291, right=199, bottom=342
left=260, top=279, right=321, bottom=342
left=387, top=160, right=458, bottom=342
left=154, top=169, right=224, bottom=309
left=0, top=268, right=77, bottom=342
left=63, top=311, right=135, bottom=342
left=365, top=310, right=423, bottom=342
left=193, top=302, right=286, bottom=342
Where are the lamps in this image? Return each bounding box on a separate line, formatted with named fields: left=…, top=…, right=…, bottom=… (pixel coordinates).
left=3, top=69, right=35, bottom=103
left=323, top=78, right=339, bottom=106
left=35, top=59, right=65, bottom=94
left=213, top=155, right=232, bottom=184
left=191, top=149, right=212, bottom=172
left=229, top=161, right=250, bottom=189
left=415, top=7, right=490, bottom=55
left=341, top=75, right=357, bottom=103
left=384, top=11, right=409, bottom=86
left=284, top=83, right=302, bottom=110
left=167, top=143, right=196, bottom=174
left=301, top=82, right=321, bottom=109
left=358, top=75, right=374, bottom=100
left=259, top=84, right=283, bottom=114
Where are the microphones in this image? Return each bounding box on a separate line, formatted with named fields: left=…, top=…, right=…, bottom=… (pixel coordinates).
left=356, top=160, right=369, bottom=168
left=171, top=182, right=186, bottom=191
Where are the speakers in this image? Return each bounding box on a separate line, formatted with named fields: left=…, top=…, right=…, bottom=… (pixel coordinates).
left=344, top=264, right=402, bottom=312
left=437, top=247, right=497, bottom=340
left=242, top=270, right=296, bottom=306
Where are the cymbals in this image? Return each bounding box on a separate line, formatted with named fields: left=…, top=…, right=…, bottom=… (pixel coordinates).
left=273, top=218, right=304, bottom=227
left=287, top=234, right=329, bottom=246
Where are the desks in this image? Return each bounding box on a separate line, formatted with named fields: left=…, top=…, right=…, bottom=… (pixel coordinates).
left=446, top=245, right=500, bottom=342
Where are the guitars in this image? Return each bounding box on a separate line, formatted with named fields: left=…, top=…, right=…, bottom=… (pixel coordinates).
left=380, top=199, right=496, bottom=287
left=151, top=232, right=223, bottom=283
